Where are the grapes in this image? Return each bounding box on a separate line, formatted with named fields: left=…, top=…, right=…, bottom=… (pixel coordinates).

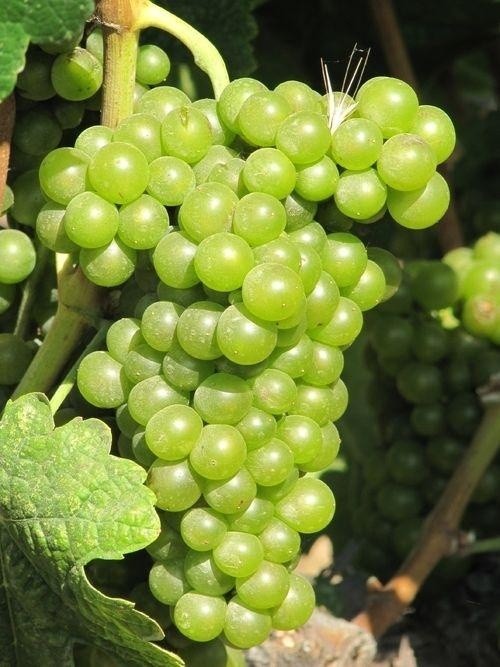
left=1, top=26, right=498, bottom=667
